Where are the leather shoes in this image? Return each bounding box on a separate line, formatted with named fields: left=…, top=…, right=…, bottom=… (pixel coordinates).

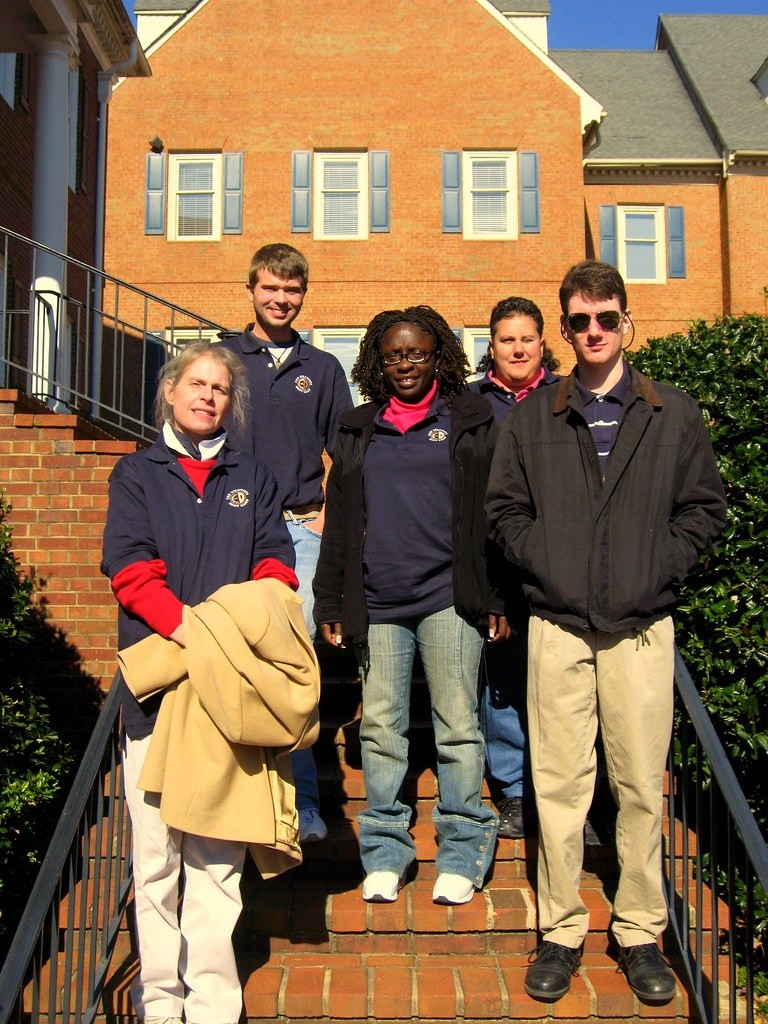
left=523, top=938, right=585, bottom=999
left=610, top=941, right=676, bottom=1001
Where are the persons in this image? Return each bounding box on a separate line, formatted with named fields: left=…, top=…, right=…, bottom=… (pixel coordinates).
left=485, top=260, right=727, bottom=999
left=311, top=305, right=512, bottom=905
left=464, top=296, right=617, bottom=842
left=100, top=345, right=299, bottom=1024
left=206, top=243, right=355, bottom=842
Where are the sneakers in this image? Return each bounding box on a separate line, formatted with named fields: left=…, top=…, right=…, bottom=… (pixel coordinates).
left=432, top=873, right=477, bottom=905
left=498, top=798, right=532, bottom=838
left=298, top=808, right=327, bottom=851
left=363, top=871, right=404, bottom=903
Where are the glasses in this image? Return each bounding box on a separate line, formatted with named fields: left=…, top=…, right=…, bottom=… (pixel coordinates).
left=566, top=311, right=622, bottom=333
left=381, top=349, right=434, bottom=365
